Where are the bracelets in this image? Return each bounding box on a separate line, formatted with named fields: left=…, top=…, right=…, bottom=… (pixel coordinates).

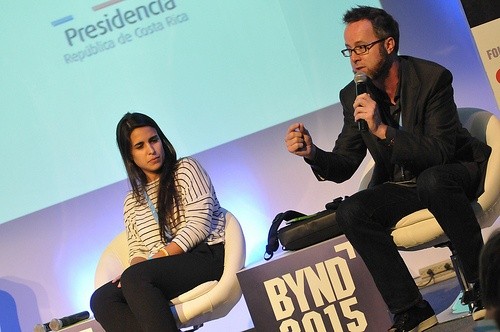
left=160, top=248, right=169, bottom=256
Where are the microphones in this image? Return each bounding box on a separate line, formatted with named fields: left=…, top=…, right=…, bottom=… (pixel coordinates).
left=34, top=311, right=90, bottom=332
left=354, top=71, right=368, bottom=132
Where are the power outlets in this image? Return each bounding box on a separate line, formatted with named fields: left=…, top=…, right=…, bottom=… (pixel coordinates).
left=419, top=258, right=455, bottom=280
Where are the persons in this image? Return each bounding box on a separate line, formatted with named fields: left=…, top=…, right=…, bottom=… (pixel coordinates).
left=90, top=112, right=225, bottom=332
left=286, top=6, right=492, bottom=332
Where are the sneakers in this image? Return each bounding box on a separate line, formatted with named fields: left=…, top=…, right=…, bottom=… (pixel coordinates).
left=386, top=301, right=438, bottom=332
left=462, top=285, right=491, bottom=320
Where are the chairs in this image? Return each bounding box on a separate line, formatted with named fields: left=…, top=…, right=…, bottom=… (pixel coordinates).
left=94, top=205, right=246, bottom=332
left=358, top=106, right=500, bottom=324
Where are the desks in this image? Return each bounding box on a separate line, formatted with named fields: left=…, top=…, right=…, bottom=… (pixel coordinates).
left=236, top=235, right=395, bottom=332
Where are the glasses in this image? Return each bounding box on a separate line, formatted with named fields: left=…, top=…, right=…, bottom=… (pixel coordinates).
left=340, top=38, right=384, bottom=57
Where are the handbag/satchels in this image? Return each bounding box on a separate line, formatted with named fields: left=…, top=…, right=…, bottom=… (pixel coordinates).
left=263, top=196, right=338, bottom=260
